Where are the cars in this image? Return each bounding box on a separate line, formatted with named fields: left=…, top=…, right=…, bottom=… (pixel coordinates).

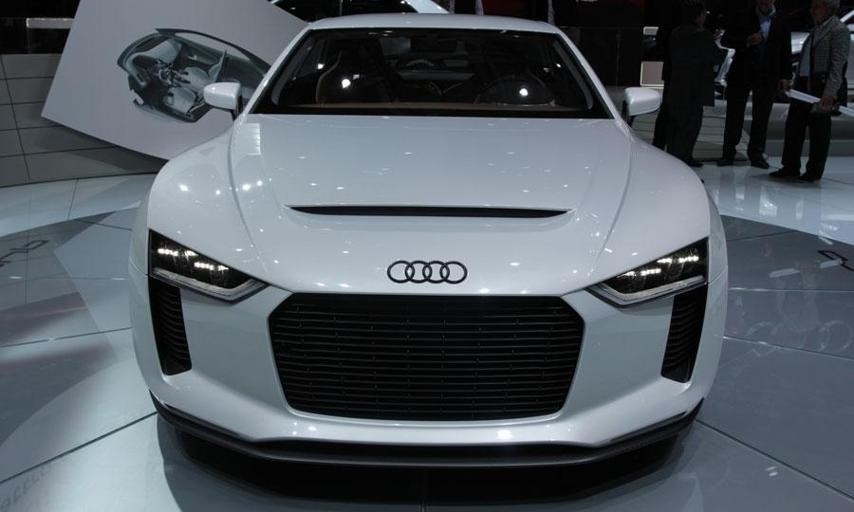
left=716, top=11, right=853, bottom=104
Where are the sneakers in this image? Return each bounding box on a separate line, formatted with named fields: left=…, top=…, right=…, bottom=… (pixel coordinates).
left=675, top=157, right=705, bottom=168
left=750, top=156, right=771, bottom=169
left=768, top=165, right=802, bottom=178
left=801, top=169, right=823, bottom=182
left=715, top=155, right=736, bottom=167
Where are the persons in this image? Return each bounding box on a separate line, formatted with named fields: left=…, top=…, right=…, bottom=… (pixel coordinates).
left=650, top=0, right=717, bottom=167
left=770, top=0, right=850, bottom=184
left=719, top=1, right=793, bottom=170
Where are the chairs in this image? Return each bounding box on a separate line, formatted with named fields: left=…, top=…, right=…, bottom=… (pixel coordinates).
left=314, top=38, right=554, bottom=106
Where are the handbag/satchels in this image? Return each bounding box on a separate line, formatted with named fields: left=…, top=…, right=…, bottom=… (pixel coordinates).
left=809, top=74, right=848, bottom=117
